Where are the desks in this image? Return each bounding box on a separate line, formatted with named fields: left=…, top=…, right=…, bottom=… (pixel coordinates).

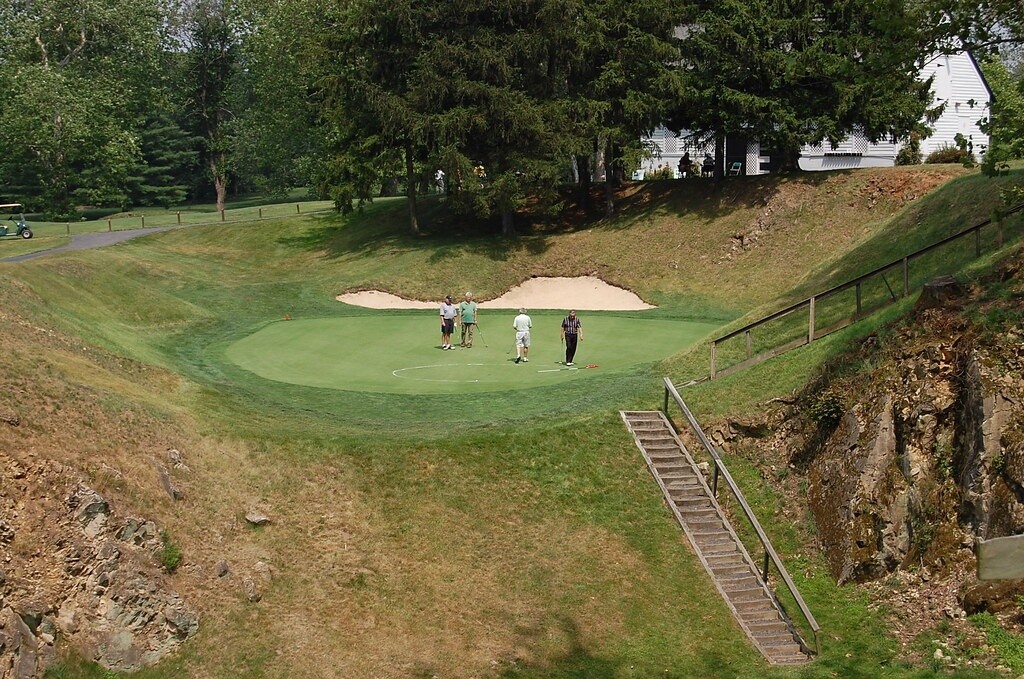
left=690, top=164, right=702, bottom=177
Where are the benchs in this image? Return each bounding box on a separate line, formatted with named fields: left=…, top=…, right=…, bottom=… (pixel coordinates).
left=0, top=224, right=8, bottom=229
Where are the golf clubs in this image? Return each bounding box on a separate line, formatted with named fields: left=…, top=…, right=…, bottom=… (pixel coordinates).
left=455, top=327, right=463, bottom=350
left=476, top=324, right=488, bottom=347
left=559, top=338, right=563, bottom=364
left=506, top=339, right=516, bottom=354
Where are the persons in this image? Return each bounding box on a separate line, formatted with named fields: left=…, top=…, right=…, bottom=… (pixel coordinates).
left=560, top=309, right=584, bottom=366
left=457, top=292, right=478, bottom=348
left=512, top=308, right=532, bottom=364
left=678, top=152, right=714, bottom=179
left=434, top=168, right=445, bottom=194
left=440, top=295, right=457, bottom=350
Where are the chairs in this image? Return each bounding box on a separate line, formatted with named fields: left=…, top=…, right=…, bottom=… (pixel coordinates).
left=730, top=162, right=742, bottom=176
left=678, top=164, right=689, bottom=178
left=702, top=160, right=718, bottom=178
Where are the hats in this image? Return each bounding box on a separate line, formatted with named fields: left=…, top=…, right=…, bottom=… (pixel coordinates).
left=519, top=307, right=528, bottom=313
left=446, top=295, right=452, bottom=299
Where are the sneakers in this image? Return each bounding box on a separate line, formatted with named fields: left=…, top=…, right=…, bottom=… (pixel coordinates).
left=515, top=356, right=521, bottom=363
left=461, top=342, right=465, bottom=347
left=467, top=343, right=471, bottom=348
left=449, top=345, right=456, bottom=350
left=571, top=362, right=574, bottom=366
left=442, top=345, right=448, bottom=349
left=523, top=358, right=528, bottom=362
left=566, top=362, right=571, bottom=366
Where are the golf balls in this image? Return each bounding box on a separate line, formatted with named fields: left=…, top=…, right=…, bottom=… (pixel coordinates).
left=476, top=380, right=478, bottom=382
